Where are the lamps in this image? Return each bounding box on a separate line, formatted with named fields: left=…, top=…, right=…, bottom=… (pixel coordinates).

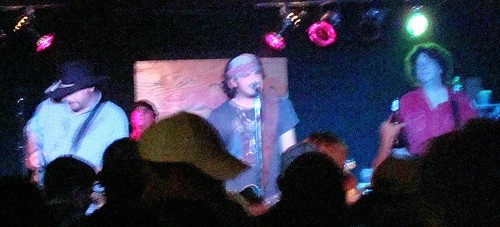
left=356, top=6, right=390, bottom=45
left=20, top=18, right=55, bottom=53
left=304, top=6, right=343, bottom=48
left=264, top=6, right=310, bottom=52
left=0, top=10, right=30, bottom=33
left=403, top=5, right=437, bottom=39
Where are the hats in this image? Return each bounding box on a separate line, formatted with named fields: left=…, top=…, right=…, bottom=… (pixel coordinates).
left=44, top=59, right=107, bottom=100
left=139, top=110, right=251, bottom=182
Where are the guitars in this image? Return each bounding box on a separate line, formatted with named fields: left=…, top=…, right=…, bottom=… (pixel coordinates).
left=240, top=158, right=357, bottom=217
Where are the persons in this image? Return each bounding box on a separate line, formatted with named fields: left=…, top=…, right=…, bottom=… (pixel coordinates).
left=127, top=100, right=159, bottom=142
left=386, top=42, right=479, bottom=161
left=22, top=60, right=130, bottom=219
left=200, top=53, right=300, bottom=208
left=0, top=112, right=500, bottom=227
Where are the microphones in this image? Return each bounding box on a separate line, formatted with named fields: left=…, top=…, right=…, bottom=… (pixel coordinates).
left=17, top=94, right=25, bottom=115
left=252, top=81, right=261, bottom=93
